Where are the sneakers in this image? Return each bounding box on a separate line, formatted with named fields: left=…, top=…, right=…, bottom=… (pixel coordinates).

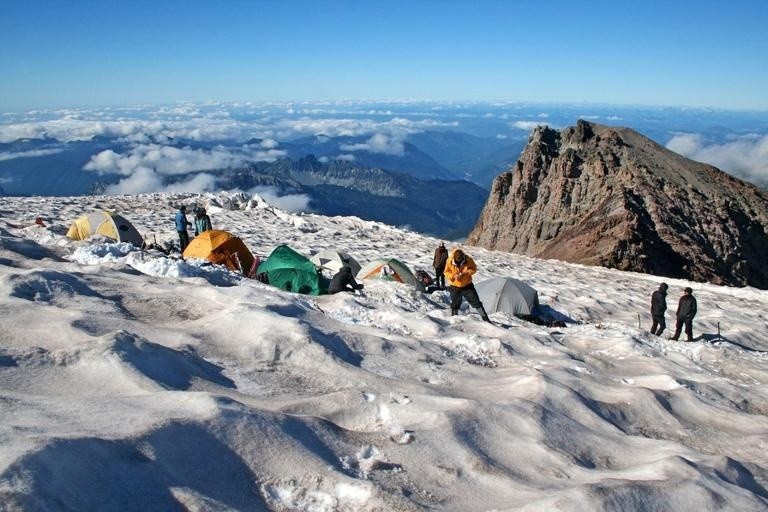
left=668, top=337, right=678, bottom=341
left=684, top=339, right=693, bottom=342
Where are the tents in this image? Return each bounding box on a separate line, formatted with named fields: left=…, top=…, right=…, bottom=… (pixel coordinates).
left=252, top=244, right=332, bottom=296
left=308, top=247, right=362, bottom=278
left=474, top=276, right=541, bottom=322
left=182, top=229, right=254, bottom=276
left=66, top=210, right=146, bottom=250
left=356, top=256, right=427, bottom=294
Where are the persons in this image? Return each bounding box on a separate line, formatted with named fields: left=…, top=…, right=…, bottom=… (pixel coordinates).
left=322, top=266, right=363, bottom=294
left=194, top=208, right=212, bottom=236
left=433, top=241, right=448, bottom=289
left=443, top=248, right=491, bottom=323
left=174, top=206, right=192, bottom=254
left=651, top=283, right=669, bottom=336
left=668, top=287, right=696, bottom=342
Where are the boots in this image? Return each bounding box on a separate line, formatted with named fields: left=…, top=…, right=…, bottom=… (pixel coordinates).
left=476, top=305, right=492, bottom=324
left=452, top=309, right=458, bottom=316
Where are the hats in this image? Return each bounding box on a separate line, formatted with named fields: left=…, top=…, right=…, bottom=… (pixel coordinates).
left=180, top=205, right=187, bottom=210
left=685, top=287, right=692, bottom=293
